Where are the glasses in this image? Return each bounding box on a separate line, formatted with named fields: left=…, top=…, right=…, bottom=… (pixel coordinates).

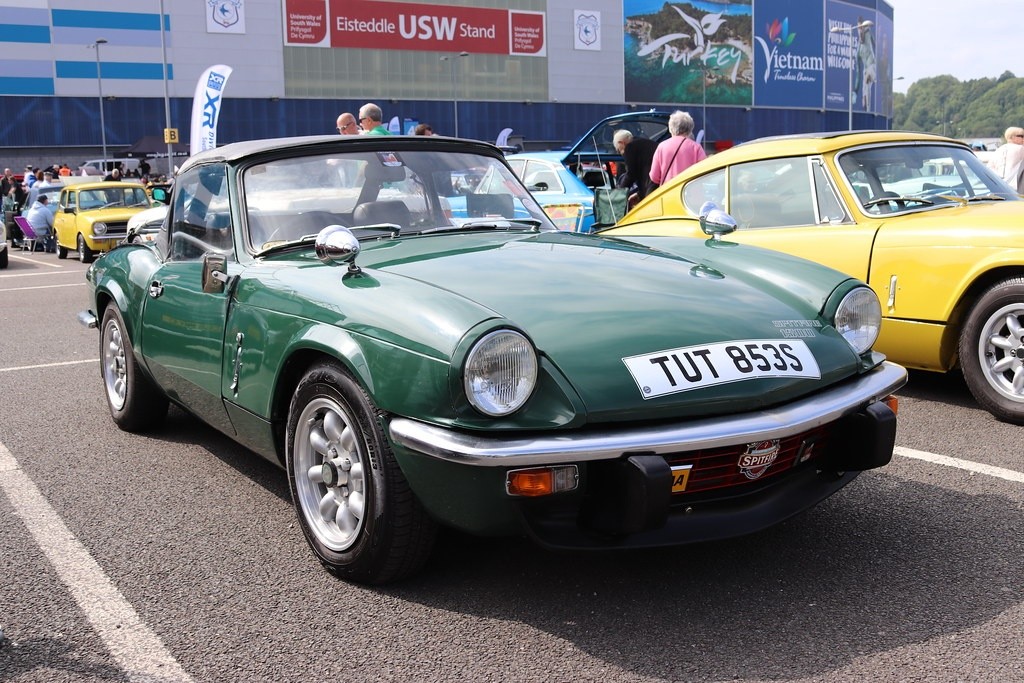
left=359, top=118, right=366, bottom=123
left=1015, top=135, right=1023, bottom=138
left=336, top=122, right=353, bottom=131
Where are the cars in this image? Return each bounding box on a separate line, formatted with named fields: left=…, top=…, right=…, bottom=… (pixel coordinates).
left=76, top=133, right=912, bottom=585
left=125, top=184, right=453, bottom=247
left=0, top=220, right=9, bottom=269
left=593, top=130, right=1024, bottom=422
left=851, top=163, right=979, bottom=202
left=925, top=142, right=997, bottom=178
left=19, top=179, right=78, bottom=251
left=51, top=180, right=161, bottom=264
left=447, top=110, right=674, bottom=235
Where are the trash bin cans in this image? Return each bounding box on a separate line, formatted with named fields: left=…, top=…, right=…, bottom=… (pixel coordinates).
left=715, top=140, right=733, bottom=153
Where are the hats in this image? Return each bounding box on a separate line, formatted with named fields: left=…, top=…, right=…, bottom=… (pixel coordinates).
left=25, top=166, right=33, bottom=170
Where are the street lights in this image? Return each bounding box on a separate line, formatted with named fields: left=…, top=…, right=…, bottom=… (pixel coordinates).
left=936, top=120, right=954, bottom=136
left=95, top=38, right=109, bottom=177
left=873, top=77, right=905, bottom=130
left=439, top=51, right=469, bottom=138
left=689, top=66, right=720, bottom=154
left=829, top=19, right=874, bottom=131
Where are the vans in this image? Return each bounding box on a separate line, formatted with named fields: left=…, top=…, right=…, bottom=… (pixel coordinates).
left=77, top=158, right=143, bottom=176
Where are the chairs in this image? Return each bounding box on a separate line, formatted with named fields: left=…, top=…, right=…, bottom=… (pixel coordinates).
left=354, top=200, right=410, bottom=233
left=6, top=211, right=23, bottom=248
left=14, top=217, right=53, bottom=252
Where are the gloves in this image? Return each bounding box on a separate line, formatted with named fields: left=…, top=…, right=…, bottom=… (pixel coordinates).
left=852, top=90, right=857, bottom=104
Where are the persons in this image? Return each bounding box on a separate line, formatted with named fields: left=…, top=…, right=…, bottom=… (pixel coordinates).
left=853, top=17, right=875, bottom=112
left=415, top=124, right=439, bottom=136
left=613, top=128, right=659, bottom=201
left=987, top=127, right=1024, bottom=189
left=326, top=102, right=410, bottom=192
left=0, top=158, right=151, bottom=253
left=648, top=109, right=708, bottom=187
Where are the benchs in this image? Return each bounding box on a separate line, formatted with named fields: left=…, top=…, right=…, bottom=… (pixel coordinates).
left=203, top=211, right=353, bottom=256
